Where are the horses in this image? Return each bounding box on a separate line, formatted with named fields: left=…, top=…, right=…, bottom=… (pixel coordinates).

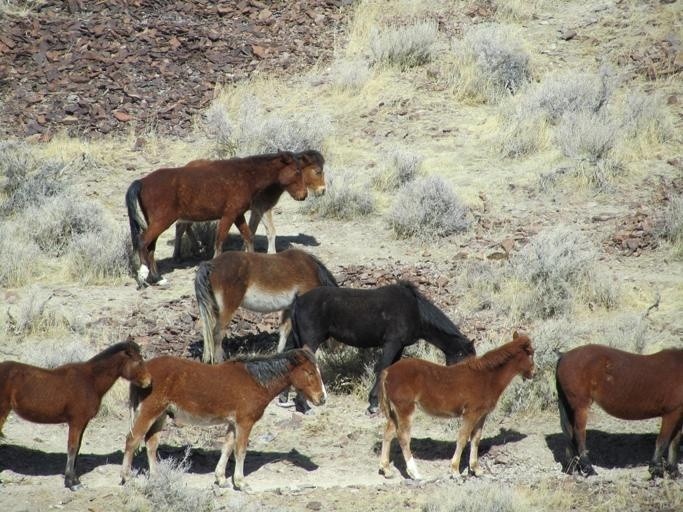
left=119, top=343, right=328, bottom=494
left=193, top=247, right=341, bottom=364
left=278, top=275, right=478, bottom=415
left=0, top=333, right=153, bottom=493
left=124, top=147, right=327, bottom=289
left=376, top=330, right=539, bottom=484
left=554, top=343, right=683, bottom=485
